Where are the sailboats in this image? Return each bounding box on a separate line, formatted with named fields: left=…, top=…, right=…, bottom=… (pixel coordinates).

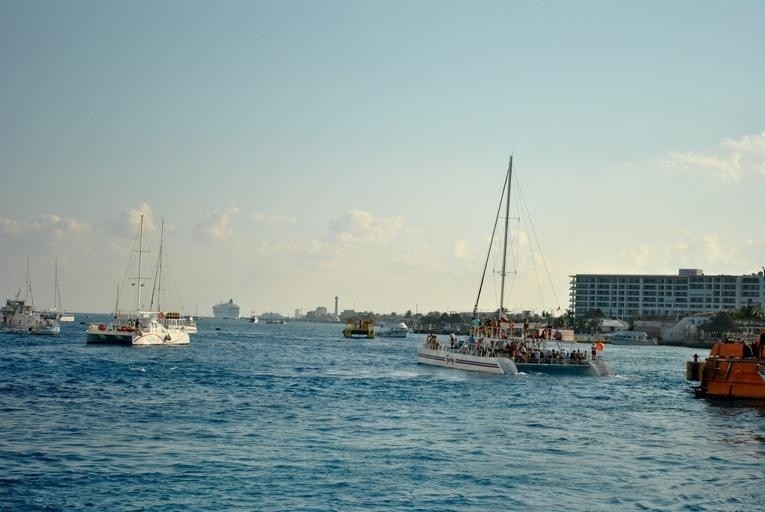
left=0, top=214, right=199, bottom=349
left=416, top=154, right=614, bottom=378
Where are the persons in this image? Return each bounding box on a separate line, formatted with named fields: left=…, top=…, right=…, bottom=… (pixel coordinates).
left=426, top=317, right=597, bottom=364
left=128, top=319, right=142, bottom=328
left=165, top=312, right=180, bottom=319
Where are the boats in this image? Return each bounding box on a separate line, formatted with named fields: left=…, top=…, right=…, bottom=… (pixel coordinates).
left=343, top=317, right=412, bottom=340
left=212, top=298, right=288, bottom=326
left=682, top=326, right=764, bottom=406
left=607, top=330, right=661, bottom=347
